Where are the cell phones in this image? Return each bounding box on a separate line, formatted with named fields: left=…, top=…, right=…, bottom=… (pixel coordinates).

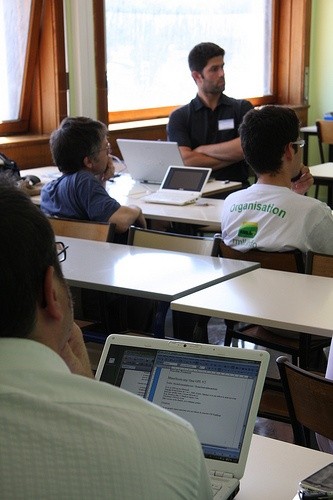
left=299, top=462, right=333, bottom=500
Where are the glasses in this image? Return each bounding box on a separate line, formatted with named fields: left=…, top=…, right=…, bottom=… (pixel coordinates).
left=280, top=140, right=305, bottom=160
left=85, top=142, right=110, bottom=156
left=38, top=241, right=69, bottom=308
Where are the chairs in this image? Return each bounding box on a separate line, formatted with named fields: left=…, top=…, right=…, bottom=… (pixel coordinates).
left=275, top=355, right=333, bottom=447
left=47, top=215, right=333, bottom=368
left=316, top=119, right=333, bottom=164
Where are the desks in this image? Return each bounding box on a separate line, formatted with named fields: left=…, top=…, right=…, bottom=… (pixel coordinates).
left=170, top=268, right=333, bottom=337
left=308, top=162, right=333, bottom=211
left=20, top=166, right=242, bottom=225
left=56, top=235, right=261, bottom=337
left=300, top=125, right=318, bottom=166
left=234, top=433, right=333, bottom=500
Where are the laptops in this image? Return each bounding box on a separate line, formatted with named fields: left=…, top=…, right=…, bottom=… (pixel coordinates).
left=143, top=165, right=212, bottom=206
left=117, top=138, right=215, bottom=182
left=94, top=334, right=270, bottom=500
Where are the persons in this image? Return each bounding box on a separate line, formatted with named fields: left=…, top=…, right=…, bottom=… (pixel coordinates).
left=222, top=104, right=333, bottom=332
left=40, top=116, right=147, bottom=232
left=0, top=172, right=211, bottom=500
left=168, top=42, right=255, bottom=200
